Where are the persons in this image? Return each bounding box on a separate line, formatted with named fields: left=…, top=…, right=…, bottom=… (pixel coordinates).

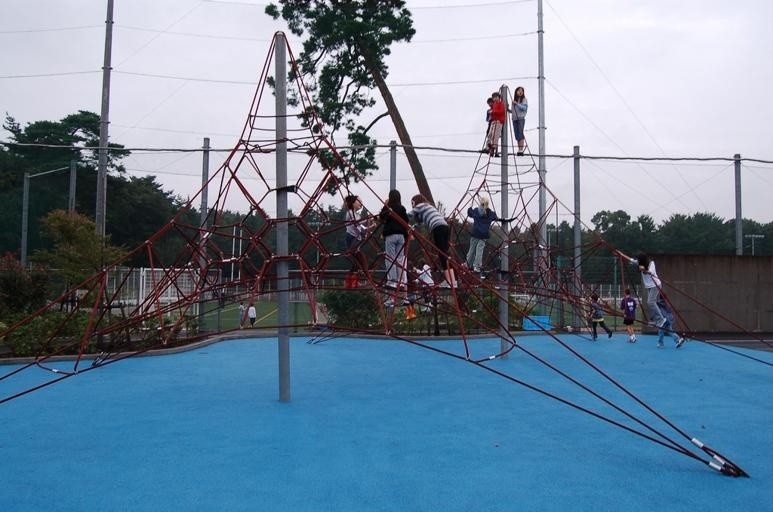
left=647, top=294, right=685, bottom=350
left=610, top=248, right=667, bottom=328
left=477, top=93, right=505, bottom=158
left=618, top=288, right=637, bottom=343
left=339, top=194, right=371, bottom=290
left=246, top=302, right=256, bottom=328
left=485, top=98, right=499, bottom=159
left=586, top=293, right=612, bottom=341
left=378, top=189, right=459, bottom=323
left=342, top=268, right=369, bottom=289
left=505, top=86, right=528, bottom=156
left=463, top=192, right=515, bottom=280
left=237, top=301, right=246, bottom=330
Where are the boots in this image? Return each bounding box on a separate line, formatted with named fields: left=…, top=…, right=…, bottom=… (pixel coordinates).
left=352, top=272, right=364, bottom=288
left=406, top=308, right=411, bottom=320
left=410, top=305, right=417, bottom=318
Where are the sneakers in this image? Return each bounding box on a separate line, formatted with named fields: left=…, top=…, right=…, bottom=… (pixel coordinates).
left=648, top=321, right=657, bottom=328
left=384, top=300, right=395, bottom=307
left=388, top=282, right=406, bottom=287
left=675, top=337, right=685, bottom=348
left=439, top=280, right=458, bottom=289
left=657, top=344, right=664, bottom=349
left=400, top=297, right=411, bottom=306
left=656, top=318, right=666, bottom=328
